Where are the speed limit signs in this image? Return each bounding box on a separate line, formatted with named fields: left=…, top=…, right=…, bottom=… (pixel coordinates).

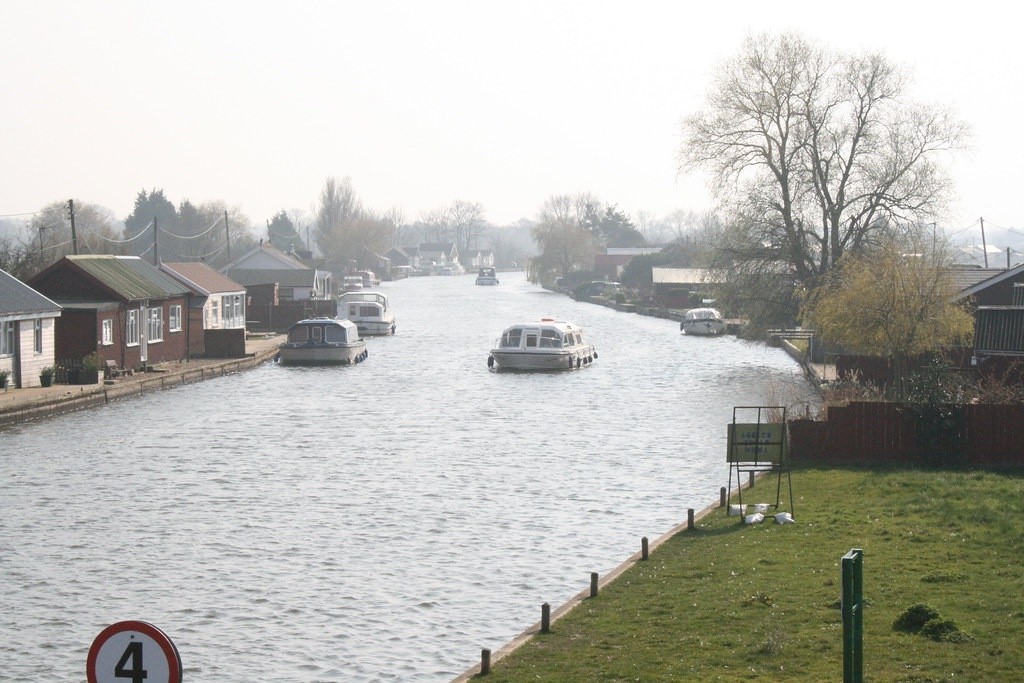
left=87, top=620, right=182, bottom=683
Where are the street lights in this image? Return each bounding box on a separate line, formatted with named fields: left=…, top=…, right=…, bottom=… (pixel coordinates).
left=39, top=226, right=46, bottom=255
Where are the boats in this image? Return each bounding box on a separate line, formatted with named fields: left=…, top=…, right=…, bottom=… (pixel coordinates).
left=683, top=308, right=726, bottom=335
left=475, top=267, right=499, bottom=285
left=344, top=276, right=363, bottom=292
left=278, top=317, right=366, bottom=365
left=333, top=292, right=395, bottom=335
left=490, top=317, right=597, bottom=369
